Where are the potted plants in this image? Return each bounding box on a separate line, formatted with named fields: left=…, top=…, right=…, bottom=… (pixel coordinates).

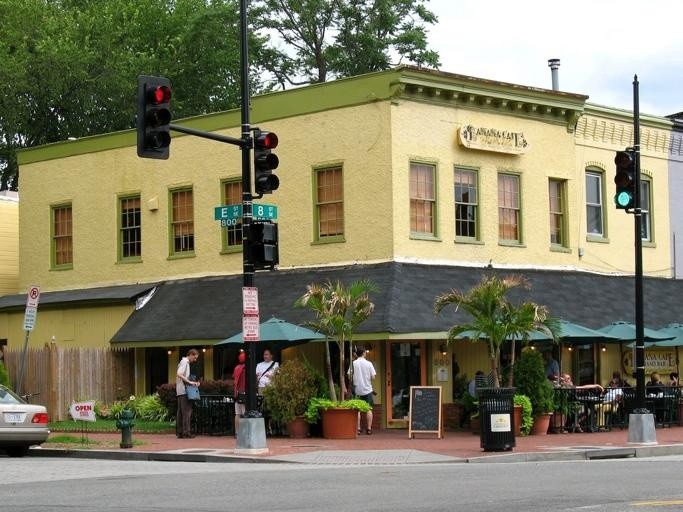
left=262, top=358, right=327, bottom=439
left=550, top=390, right=568, bottom=434
left=455, top=381, right=495, bottom=435
left=433, top=271, right=564, bottom=437
left=295, top=278, right=382, bottom=440
left=514, top=346, right=555, bottom=437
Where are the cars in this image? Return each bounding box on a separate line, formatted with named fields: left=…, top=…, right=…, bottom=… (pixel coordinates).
left=0, top=384, right=48, bottom=457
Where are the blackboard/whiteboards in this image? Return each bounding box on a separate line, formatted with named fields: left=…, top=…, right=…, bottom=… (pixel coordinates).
left=409, top=385, right=442, bottom=433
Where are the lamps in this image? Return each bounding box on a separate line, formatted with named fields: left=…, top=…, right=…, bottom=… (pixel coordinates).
left=440, top=344, right=448, bottom=356
left=601, top=344, right=606, bottom=352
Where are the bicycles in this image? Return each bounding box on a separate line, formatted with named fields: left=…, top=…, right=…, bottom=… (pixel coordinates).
left=17, top=391, right=40, bottom=404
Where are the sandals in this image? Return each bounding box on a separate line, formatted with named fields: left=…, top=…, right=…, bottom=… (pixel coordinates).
left=358, top=430, right=372, bottom=435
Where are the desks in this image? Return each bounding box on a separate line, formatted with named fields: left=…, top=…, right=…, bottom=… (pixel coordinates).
left=579, top=390, right=683, bottom=432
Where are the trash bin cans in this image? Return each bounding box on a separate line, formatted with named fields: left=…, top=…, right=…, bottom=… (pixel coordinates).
left=476, top=387, right=518, bottom=452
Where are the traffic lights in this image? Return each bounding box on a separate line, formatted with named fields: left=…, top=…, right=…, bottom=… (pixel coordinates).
left=613, top=151, right=636, bottom=210
left=253, top=129, right=279, bottom=194
left=137, top=75, right=171, bottom=159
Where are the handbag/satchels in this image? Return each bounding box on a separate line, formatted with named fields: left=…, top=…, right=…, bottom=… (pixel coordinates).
left=186, top=384, right=200, bottom=400
left=238, top=394, right=246, bottom=404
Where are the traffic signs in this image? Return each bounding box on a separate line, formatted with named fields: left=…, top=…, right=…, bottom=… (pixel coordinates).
left=214, top=204, right=242, bottom=227
left=69, top=398, right=96, bottom=424
left=251, top=203, right=277, bottom=219
left=23, top=308, right=37, bottom=331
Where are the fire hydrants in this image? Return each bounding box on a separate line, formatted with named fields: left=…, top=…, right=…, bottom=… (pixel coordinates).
left=115, top=406, right=135, bottom=449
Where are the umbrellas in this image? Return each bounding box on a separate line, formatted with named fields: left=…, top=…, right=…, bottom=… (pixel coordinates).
left=212, top=313, right=336, bottom=367
left=457, top=314, right=682, bottom=379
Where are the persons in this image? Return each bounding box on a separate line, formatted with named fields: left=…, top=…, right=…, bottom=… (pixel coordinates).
left=334, top=345, right=354, bottom=399
left=232, top=350, right=245, bottom=438
left=468, top=370, right=487, bottom=401
left=542, top=348, right=559, bottom=379
left=174, top=349, right=200, bottom=438
left=546, top=369, right=683, bottom=434
left=345, top=346, right=376, bottom=435
left=255, top=349, right=281, bottom=435
